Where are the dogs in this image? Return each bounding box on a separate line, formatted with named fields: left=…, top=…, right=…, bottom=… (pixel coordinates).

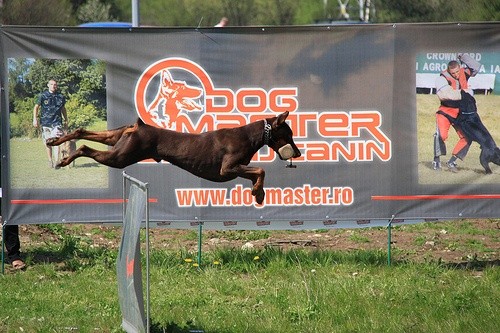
left=435, top=89, right=500, bottom=175
left=46, top=110, right=301, bottom=205
left=54, top=126, right=77, bottom=167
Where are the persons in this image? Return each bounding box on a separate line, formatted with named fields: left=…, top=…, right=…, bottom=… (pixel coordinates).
left=432, top=53, right=481, bottom=173
left=33, top=80, right=68, bottom=169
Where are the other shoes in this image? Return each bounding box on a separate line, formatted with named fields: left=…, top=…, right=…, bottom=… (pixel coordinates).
left=12, top=260, right=25, bottom=269
left=54, top=161, right=60, bottom=169
left=445, top=161, right=457, bottom=173
left=432, top=156, right=441, bottom=169
left=48, top=161, right=53, bottom=169
